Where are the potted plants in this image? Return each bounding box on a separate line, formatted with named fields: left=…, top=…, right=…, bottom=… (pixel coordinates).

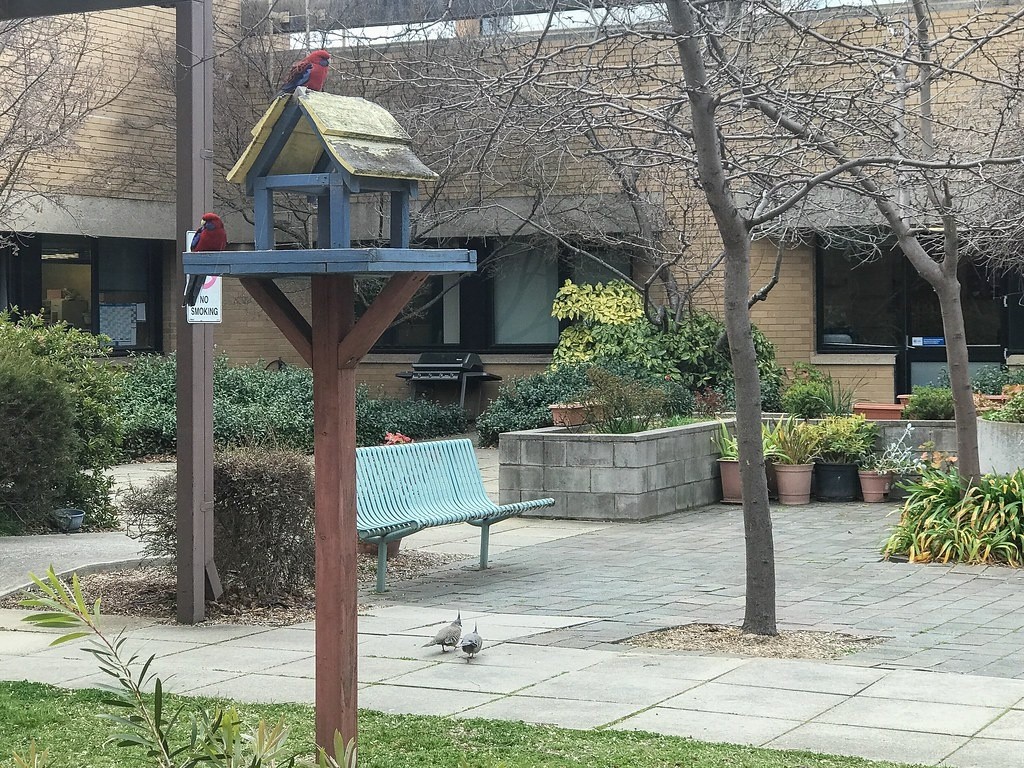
left=849, top=423, right=925, bottom=501
left=814, top=416, right=865, bottom=501
left=711, top=413, right=767, bottom=505
left=773, top=413, right=822, bottom=504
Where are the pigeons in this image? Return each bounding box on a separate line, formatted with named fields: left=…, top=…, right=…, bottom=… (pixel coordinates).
left=460, top=621, right=482, bottom=664
left=421, top=613, right=463, bottom=654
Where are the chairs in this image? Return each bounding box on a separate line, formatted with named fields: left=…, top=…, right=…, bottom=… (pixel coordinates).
left=354, top=438, right=556, bottom=596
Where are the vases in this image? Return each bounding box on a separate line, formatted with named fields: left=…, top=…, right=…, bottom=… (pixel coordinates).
left=548, top=403, right=587, bottom=427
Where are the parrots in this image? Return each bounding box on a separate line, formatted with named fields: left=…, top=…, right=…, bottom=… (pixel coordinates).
left=182, top=213, right=227, bottom=307
left=268, top=50, right=330, bottom=104
